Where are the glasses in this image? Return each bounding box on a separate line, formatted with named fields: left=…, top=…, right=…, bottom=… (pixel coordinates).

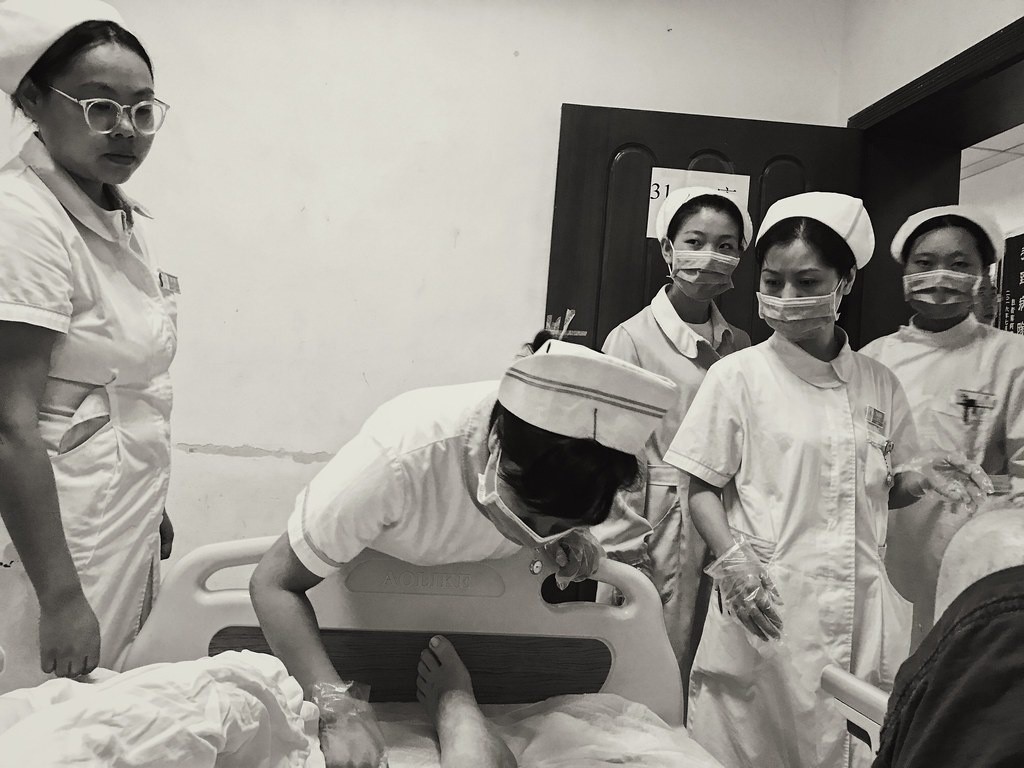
left=47, top=85, right=171, bottom=135
left=973, top=293, right=1005, bottom=304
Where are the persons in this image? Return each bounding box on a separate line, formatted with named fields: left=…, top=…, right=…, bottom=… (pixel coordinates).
left=592, top=186, right=755, bottom=707
left=246, top=330, right=680, bottom=768
left=855, top=203, right=1024, bottom=693
left=886, top=508, right=1024, bottom=768
left=418, top=630, right=521, bottom=768
left=0, top=0, right=184, bottom=696
left=665, top=190, right=993, bottom=767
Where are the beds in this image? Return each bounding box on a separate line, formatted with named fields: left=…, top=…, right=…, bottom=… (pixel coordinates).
left=1, top=533, right=893, bottom=768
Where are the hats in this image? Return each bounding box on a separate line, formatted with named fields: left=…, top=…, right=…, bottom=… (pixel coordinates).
left=754, top=190, right=875, bottom=271
left=656, top=186, right=753, bottom=253
left=0, top=0, right=128, bottom=94
left=890, top=205, right=1005, bottom=268
left=496, top=339, right=679, bottom=456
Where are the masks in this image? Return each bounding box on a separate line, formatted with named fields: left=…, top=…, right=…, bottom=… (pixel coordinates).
left=477, top=441, right=591, bottom=547
left=756, top=276, right=846, bottom=342
left=668, top=239, right=740, bottom=304
left=903, top=269, right=983, bottom=320
